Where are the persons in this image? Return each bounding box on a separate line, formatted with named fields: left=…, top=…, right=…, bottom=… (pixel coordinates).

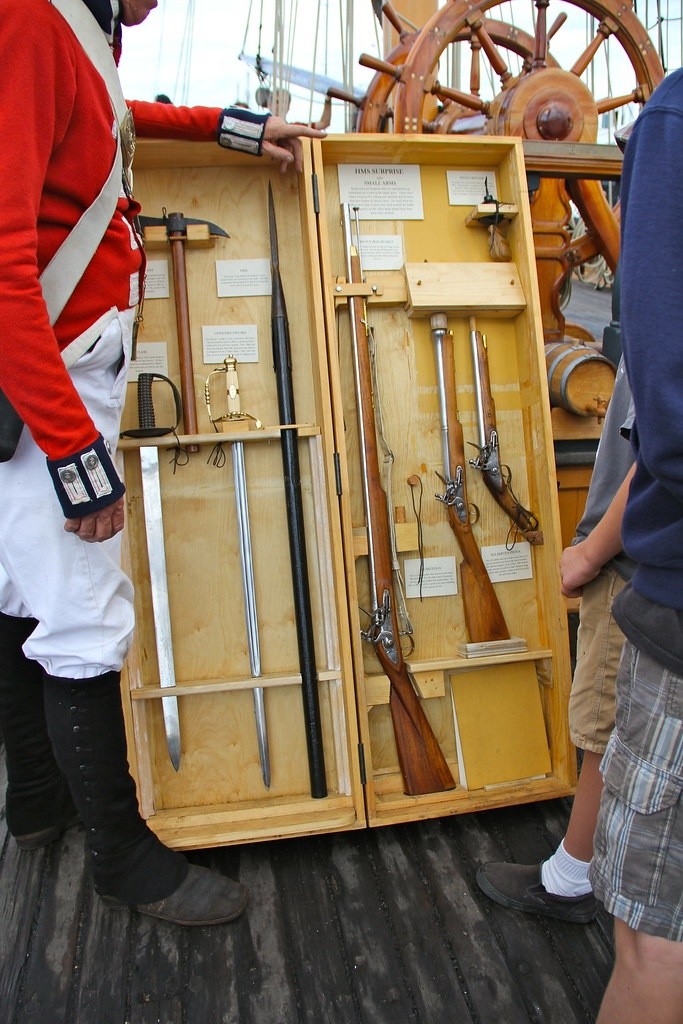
left=0, top=0, right=333, bottom=930
left=585, top=63, right=683, bottom=1024
left=472, top=346, right=647, bottom=926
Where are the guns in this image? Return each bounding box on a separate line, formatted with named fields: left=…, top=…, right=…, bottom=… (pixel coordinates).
left=341, top=201, right=457, bottom=793
left=465, top=318, right=546, bottom=544
left=428, top=313, right=512, bottom=643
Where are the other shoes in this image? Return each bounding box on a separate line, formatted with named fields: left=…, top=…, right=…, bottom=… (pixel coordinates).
left=17, top=817, right=83, bottom=852
left=93, top=857, right=249, bottom=925
left=476, top=861, right=598, bottom=925
